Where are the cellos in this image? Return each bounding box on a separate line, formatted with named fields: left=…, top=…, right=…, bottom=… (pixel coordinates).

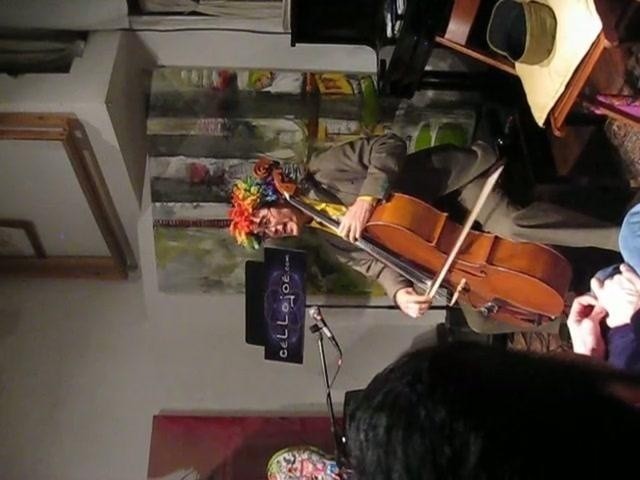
left=253, top=159, right=574, bottom=329
